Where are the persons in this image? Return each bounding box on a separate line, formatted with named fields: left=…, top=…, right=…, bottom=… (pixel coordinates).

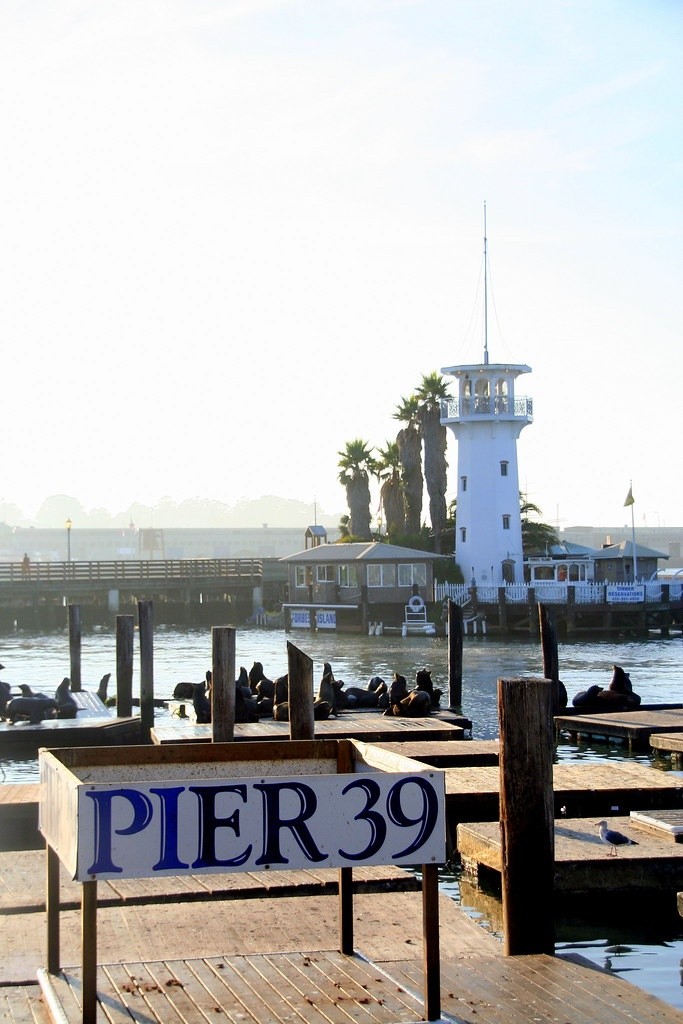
left=23, top=553, right=30, bottom=570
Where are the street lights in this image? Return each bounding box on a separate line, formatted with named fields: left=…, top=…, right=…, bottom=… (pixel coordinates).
left=64, top=517, right=73, bottom=580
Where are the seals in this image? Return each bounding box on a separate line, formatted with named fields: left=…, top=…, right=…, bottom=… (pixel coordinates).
left=0, top=664, right=641, bottom=725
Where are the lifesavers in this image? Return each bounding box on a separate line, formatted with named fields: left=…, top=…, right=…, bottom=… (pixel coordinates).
left=409, top=596, right=424, bottom=611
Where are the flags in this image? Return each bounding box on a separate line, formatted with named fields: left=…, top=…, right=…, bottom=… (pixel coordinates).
left=624, top=488, right=634, bottom=507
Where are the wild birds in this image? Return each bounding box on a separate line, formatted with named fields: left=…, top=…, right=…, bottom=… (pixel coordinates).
left=595, top=819, right=639, bottom=857
